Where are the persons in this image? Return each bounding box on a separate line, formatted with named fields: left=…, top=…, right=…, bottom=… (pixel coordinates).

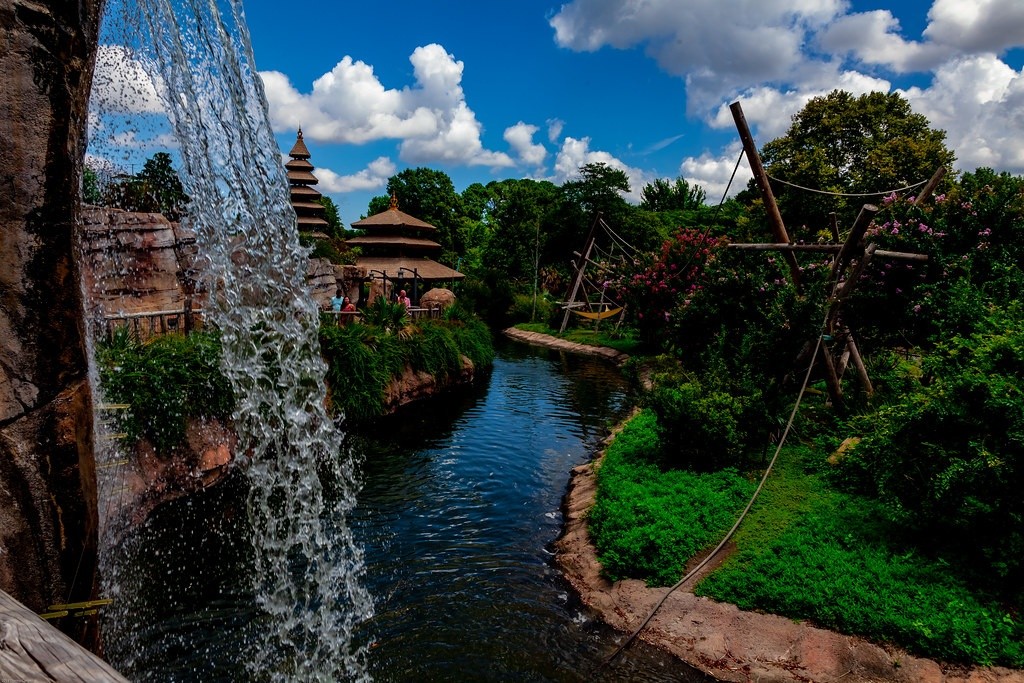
left=397, top=289, right=412, bottom=318
left=323, top=288, right=355, bottom=333
